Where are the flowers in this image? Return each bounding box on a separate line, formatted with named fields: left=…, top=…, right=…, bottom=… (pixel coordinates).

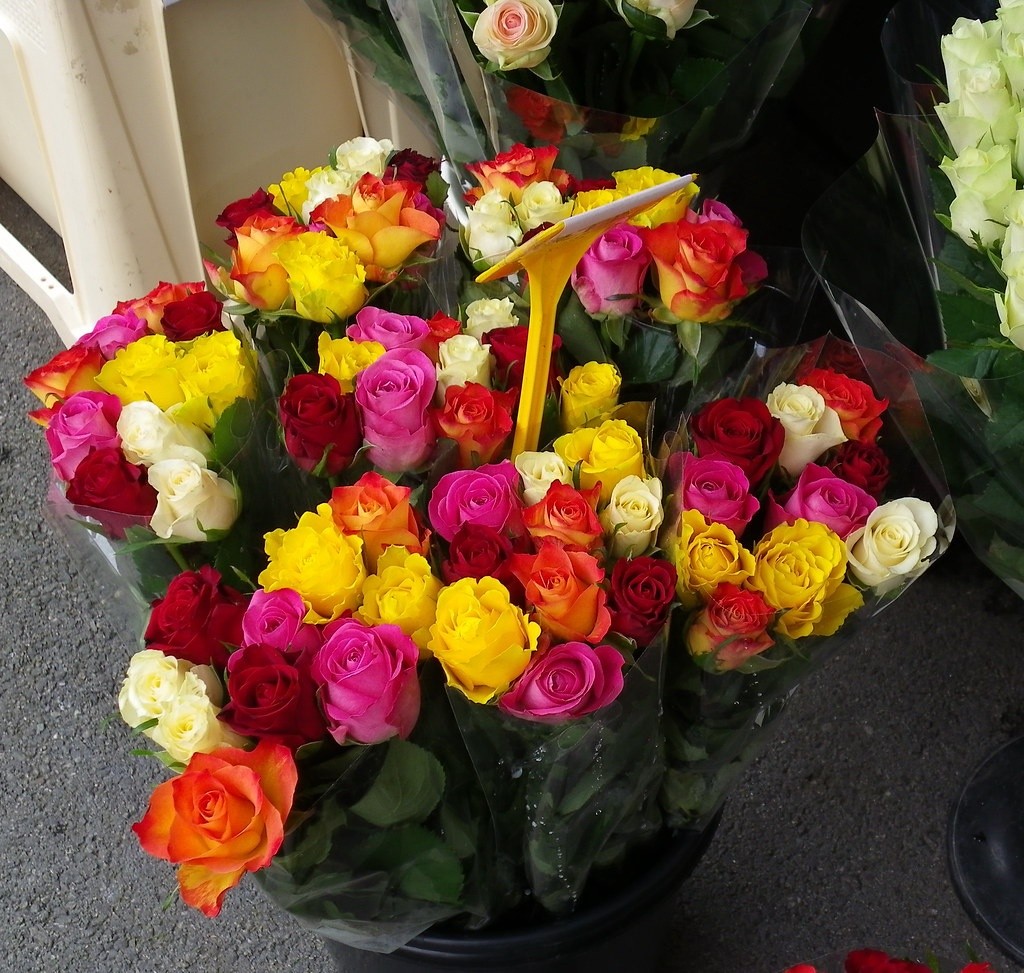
left=22, top=0, right=1024, bottom=950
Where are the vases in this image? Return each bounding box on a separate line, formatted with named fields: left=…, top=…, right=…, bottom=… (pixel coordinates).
left=323, top=797, right=728, bottom=973
